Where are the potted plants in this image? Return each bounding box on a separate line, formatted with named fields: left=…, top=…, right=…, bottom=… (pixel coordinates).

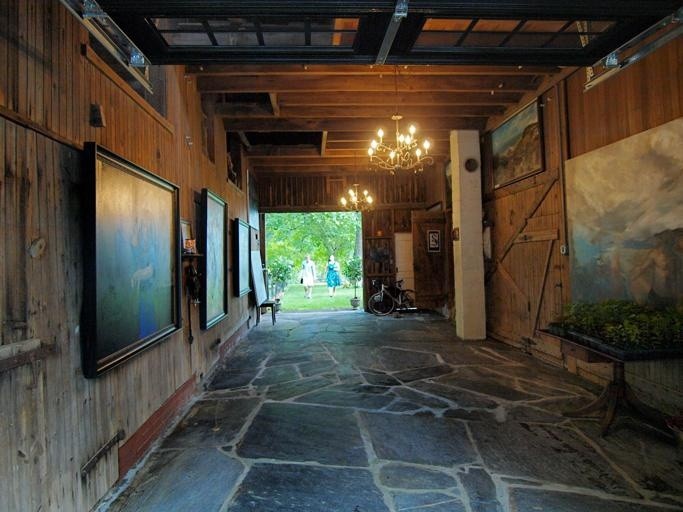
left=344, top=257, right=362, bottom=310
left=548, top=297, right=682, bottom=361
left=268, top=260, right=290, bottom=311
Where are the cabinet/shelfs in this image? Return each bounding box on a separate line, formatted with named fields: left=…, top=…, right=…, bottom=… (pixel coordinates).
left=363, top=236, right=396, bottom=278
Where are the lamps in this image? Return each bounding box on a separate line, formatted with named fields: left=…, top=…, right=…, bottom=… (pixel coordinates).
left=361, top=65, right=439, bottom=175
left=334, top=172, right=377, bottom=214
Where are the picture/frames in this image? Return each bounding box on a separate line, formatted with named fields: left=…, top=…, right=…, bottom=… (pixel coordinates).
left=201, top=189, right=228, bottom=332
left=488, top=95, right=545, bottom=192
left=235, top=219, right=250, bottom=297
left=83, top=140, right=182, bottom=381
left=250, top=250, right=268, bottom=306
left=426, top=231, right=440, bottom=252
left=180, top=218, right=194, bottom=254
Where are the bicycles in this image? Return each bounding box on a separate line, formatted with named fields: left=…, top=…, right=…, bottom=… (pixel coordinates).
left=368, top=279, right=416, bottom=316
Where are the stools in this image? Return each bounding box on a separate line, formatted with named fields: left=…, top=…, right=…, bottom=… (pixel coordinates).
left=256, top=303, right=274, bottom=326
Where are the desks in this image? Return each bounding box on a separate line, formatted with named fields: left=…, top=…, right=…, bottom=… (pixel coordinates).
left=536, top=329, right=683, bottom=445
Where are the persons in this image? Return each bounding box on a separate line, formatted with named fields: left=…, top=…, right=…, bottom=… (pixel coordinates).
left=299, top=254, right=316, bottom=299
left=124, top=225, right=159, bottom=342
left=322, top=254, right=342, bottom=298
